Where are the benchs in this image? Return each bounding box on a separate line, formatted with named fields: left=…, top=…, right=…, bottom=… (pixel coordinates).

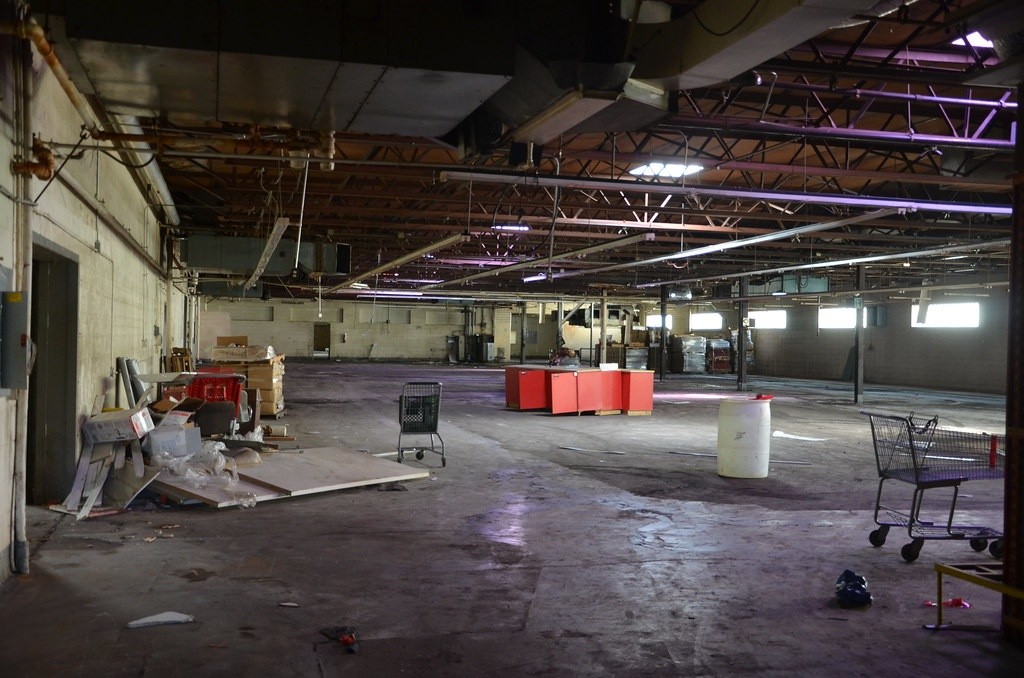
left=922, top=561, right=1024, bottom=630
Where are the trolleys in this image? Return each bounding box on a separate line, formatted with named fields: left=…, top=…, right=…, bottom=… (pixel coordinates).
left=397, top=381, right=446, bottom=467
left=860, top=410, right=1006, bottom=561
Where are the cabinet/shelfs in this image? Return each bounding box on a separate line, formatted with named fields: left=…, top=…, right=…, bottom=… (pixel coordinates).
left=501, top=364, right=655, bottom=417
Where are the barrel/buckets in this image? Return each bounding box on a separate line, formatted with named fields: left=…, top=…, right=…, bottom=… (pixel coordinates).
left=718, top=395, right=771, bottom=478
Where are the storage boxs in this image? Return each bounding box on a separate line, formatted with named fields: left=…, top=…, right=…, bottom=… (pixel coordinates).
left=82, top=342, right=286, bottom=465
